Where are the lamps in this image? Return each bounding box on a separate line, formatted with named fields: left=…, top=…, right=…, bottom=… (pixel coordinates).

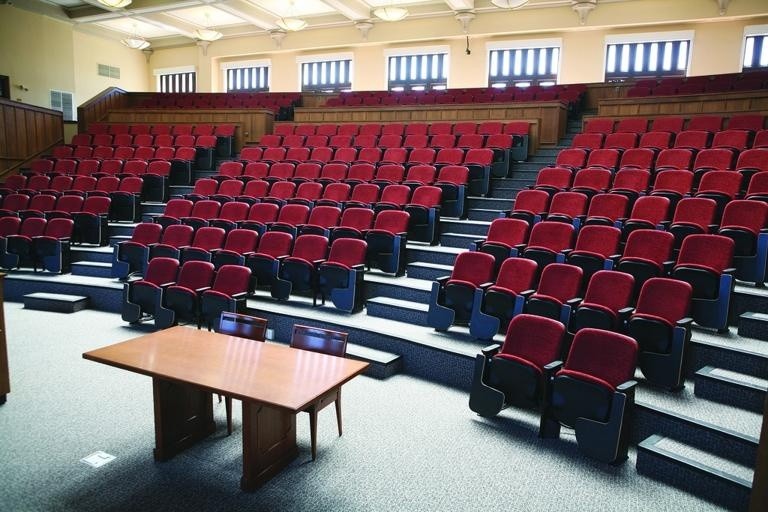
left=274, top=3, right=308, bottom=32
left=374, top=1, right=412, bottom=21
left=121, top=22, right=151, bottom=49
left=194, top=14, right=222, bottom=42
